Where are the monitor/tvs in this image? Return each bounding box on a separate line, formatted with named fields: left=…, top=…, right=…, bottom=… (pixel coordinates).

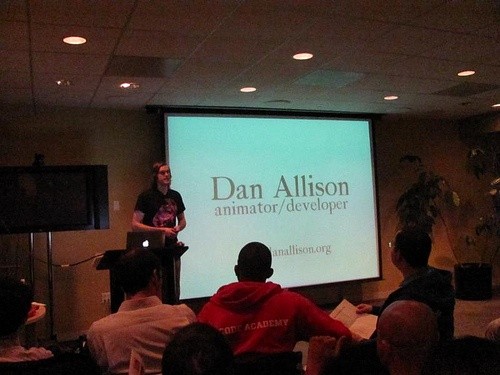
left=0, top=165, right=109, bottom=234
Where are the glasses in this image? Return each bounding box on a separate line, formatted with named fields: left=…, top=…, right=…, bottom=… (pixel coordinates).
left=388, top=240, right=398, bottom=247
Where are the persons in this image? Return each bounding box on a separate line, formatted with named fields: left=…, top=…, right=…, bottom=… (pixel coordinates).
left=0, top=268, right=54, bottom=362
left=88, top=246, right=198, bottom=375
left=195, top=242, right=371, bottom=356
left=356, top=225, right=456, bottom=344
left=129, top=162, right=186, bottom=300
left=162, top=300, right=500, bottom=374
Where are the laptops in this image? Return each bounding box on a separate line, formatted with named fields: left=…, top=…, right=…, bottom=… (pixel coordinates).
left=126, top=230, right=165, bottom=249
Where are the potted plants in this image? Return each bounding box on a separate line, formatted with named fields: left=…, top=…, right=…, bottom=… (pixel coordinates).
left=394, top=129, right=500, bottom=300
